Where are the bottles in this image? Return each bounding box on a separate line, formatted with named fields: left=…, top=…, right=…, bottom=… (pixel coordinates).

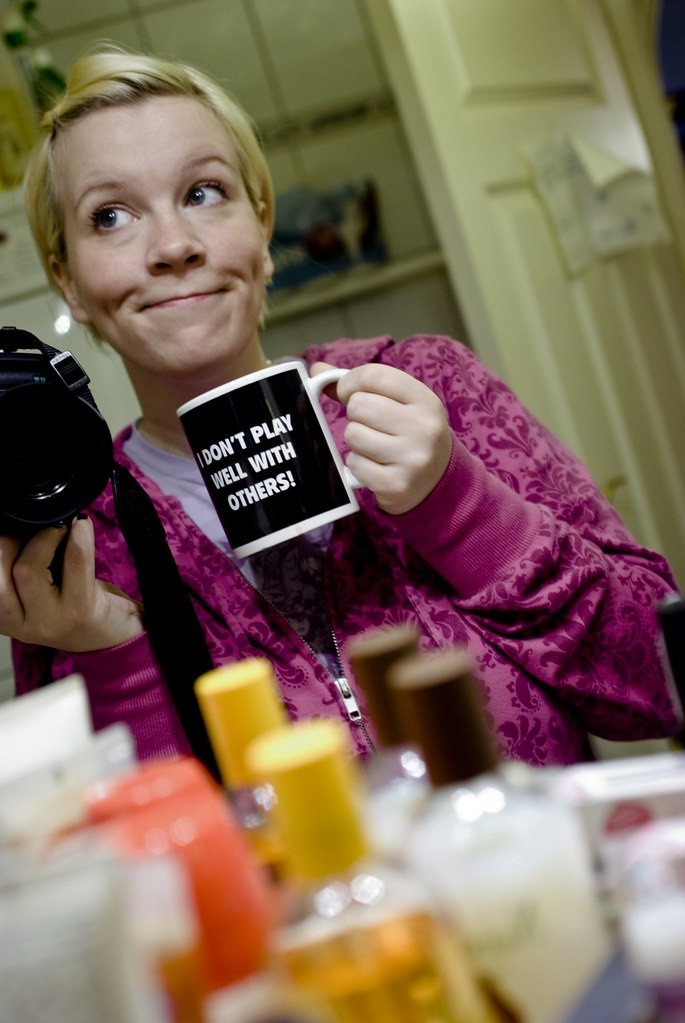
left=395, top=655, right=641, bottom=1023
left=194, top=660, right=290, bottom=829
left=345, top=626, right=425, bottom=841
left=245, top=719, right=491, bottom=1023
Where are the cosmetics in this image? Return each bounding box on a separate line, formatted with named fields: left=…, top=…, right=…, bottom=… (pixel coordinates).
left=0, top=656, right=685, bottom=1023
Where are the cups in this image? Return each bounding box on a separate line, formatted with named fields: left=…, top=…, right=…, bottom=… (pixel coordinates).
left=177, top=360, right=366, bottom=560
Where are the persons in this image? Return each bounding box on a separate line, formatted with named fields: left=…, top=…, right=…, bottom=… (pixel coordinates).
left=0, top=55, right=685, bottom=784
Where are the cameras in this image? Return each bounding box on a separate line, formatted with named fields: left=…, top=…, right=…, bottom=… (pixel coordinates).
left=0, top=352, right=113, bottom=527
